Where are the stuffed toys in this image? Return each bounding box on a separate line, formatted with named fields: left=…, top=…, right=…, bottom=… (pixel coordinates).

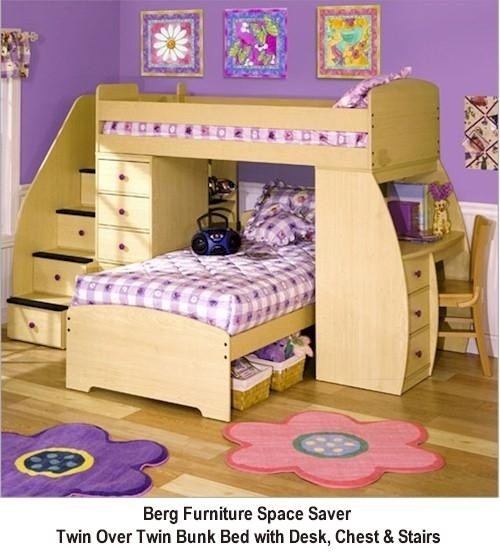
left=256, top=331, right=313, bottom=361
left=430, top=182, right=452, bottom=238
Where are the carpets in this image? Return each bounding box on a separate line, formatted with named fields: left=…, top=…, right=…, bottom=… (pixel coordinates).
left=220, top=408, right=449, bottom=491
left=1, top=421, right=170, bottom=497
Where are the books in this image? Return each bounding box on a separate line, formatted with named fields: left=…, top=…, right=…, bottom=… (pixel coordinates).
left=387, top=199, right=421, bottom=237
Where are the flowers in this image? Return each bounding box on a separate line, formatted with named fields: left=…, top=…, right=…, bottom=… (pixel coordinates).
left=151, top=21, right=192, bottom=65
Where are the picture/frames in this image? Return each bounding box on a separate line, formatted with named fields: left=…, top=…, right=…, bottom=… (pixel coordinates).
left=222, top=7, right=289, bottom=80
left=138, top=8, right=206, bottom=79
left=315, top=4, right=381, bottom=80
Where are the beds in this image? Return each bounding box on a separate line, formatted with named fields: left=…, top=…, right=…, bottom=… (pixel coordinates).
left=96, top=77, right=442, bottom=184
left=63, top=233, right=316, bottom=424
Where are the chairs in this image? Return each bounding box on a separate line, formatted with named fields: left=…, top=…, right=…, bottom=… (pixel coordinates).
left=439, top=213, right=497, bottom=378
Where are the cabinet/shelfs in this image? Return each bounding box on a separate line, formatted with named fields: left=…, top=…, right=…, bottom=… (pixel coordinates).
left=95, top=152, right=210, bottom=271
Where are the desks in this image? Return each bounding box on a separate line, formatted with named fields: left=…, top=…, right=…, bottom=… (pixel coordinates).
left=314, top=230, right=465, bottom=397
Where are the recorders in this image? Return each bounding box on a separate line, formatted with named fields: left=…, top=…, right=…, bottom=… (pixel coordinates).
left=192, top=212, right=240, bottom=254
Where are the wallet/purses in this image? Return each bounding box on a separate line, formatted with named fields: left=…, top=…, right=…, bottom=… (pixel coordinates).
left=230, top=361, right=273, bottom=411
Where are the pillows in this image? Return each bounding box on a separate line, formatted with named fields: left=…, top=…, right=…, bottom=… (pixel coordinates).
left=249, top=177, right=316, bottom=225
left=246, top=210, right=316, bottom=247
left=328, top=64, right=414, bottom=108
